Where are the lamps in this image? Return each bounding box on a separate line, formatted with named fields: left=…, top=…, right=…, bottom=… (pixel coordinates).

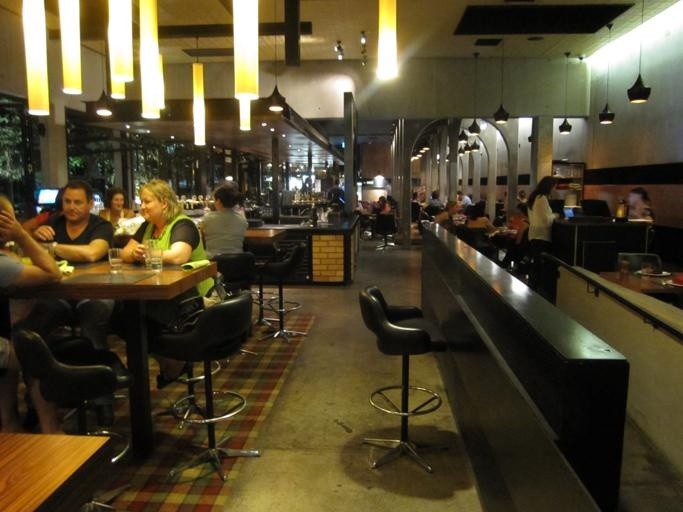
left=627, top=1, right=651, bottom=104
left=598, top=24, right=616, bottom=125
left=559, top=52, right=572, bottom=135
left=494, top=40, right=509, bottom=124
left=409, top=53, right=482, bottom=167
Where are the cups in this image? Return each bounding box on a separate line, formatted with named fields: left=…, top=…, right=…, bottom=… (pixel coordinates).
left=108, top=248, right=123, bottom=267
left=641, top=262, right=655, bottom=272
left=144, top=239, right=163, bottom=272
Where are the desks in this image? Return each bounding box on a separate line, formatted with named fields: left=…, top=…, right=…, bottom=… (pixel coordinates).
left=0, top=197, right=360, bottom=512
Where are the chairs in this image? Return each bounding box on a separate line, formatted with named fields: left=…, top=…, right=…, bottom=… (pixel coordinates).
left=358, top=284, right=448, bottom=474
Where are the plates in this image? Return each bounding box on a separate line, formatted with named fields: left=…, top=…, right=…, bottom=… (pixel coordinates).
left=666, top=280, right=683, bottom=287
left=636, top=269, right=672, bottom=277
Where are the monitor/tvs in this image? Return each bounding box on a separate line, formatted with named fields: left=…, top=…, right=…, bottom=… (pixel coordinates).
left=36, top=187, right=59, bottom=205
left=580, top=199, right=611, bottom=216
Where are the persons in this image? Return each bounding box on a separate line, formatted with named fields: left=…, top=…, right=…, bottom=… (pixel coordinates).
left=1, top=178, right=215, bottom=435
left=328, top=176, right=527, bottom=269
left=198, top=180, right=250, bottom=261
left=527, top=176, right=560, bottom=274
left=625, top=187, right=655, bottom=222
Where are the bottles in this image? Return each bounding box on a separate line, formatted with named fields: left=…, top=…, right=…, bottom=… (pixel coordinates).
left=620, top=256, right=631, bottom=282
